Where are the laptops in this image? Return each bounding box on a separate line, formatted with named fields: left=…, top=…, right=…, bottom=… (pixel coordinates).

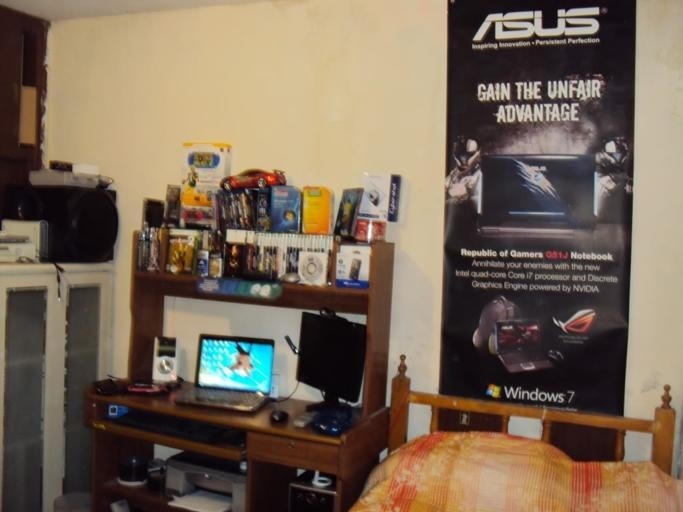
left=495, top=319, right=553, bottom=374
left=173, top=334, right=275, bottom=416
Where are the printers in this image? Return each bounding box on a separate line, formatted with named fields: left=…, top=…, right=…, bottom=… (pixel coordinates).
left=164, top=450, right=246, bottom=512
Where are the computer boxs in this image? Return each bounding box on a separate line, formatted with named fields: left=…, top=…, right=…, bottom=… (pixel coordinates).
left=288, top=470, right=336, bottom=512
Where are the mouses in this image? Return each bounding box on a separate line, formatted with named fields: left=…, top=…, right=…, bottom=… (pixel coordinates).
left=269, top=409, right=288, bottom=426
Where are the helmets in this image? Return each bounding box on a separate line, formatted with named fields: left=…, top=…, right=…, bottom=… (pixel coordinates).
left=473, top=297, right=523, bottom=354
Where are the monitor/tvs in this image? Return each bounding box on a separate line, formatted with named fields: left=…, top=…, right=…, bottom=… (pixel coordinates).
left=480, top=152, right=596, bottom=233
left=295, top=313, right=368, bottom=410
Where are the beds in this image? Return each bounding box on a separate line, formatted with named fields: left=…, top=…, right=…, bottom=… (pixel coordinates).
left=350, top=353, right=683, bottom=512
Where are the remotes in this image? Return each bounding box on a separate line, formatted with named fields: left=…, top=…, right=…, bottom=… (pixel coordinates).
left=293, top=412, right=315, bottom=428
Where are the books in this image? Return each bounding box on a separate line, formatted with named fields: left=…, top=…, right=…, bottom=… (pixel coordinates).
left=269, top=184, right=300, bottom=234
left=302, top=185, right=332, bottom=234
left=333, top=186, right=365, bottom=238
left=225, top=229, right=334, bottom=283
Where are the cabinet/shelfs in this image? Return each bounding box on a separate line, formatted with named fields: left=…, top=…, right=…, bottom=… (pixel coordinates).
left=0, top=261, right=116, bottom=512
left=83, top=229, right=395, bottom=512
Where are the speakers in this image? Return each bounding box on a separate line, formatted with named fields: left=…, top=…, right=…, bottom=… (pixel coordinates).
left=151, top=336, right=177, bottom=384
